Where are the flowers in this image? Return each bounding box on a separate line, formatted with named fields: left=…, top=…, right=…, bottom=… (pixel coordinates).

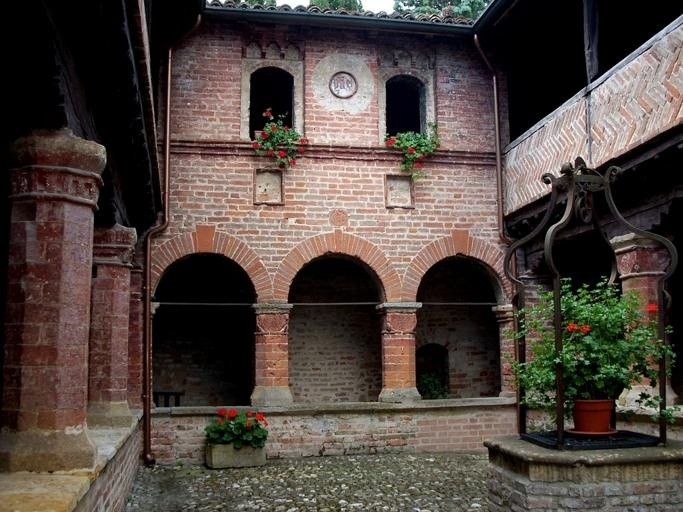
left=251, top=107, right=307, bottom=170
left=205, top=408, right=268, bottom=450
left=384, top=131, right=439, bottom=178
left=506, top=276, right=683, bottom=433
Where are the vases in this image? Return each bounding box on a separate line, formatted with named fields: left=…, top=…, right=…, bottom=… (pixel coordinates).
left=572, top=400, right=611, bottom=433
left=206, top=443, right=265, bottom=468
